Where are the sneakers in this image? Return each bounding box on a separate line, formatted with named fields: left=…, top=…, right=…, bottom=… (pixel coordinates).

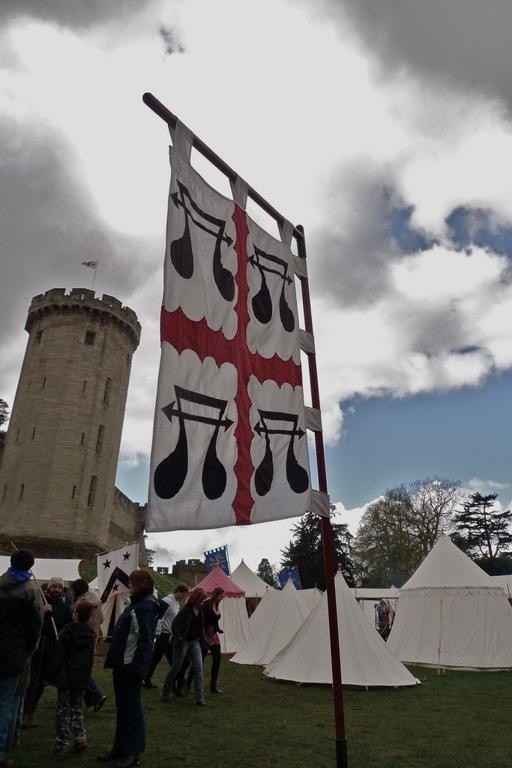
left=93, top=695, right=107, bottom=713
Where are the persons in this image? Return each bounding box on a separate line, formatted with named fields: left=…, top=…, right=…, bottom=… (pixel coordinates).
left=18, top=583, right=72, bottom=729
left=380, top=599, right=393, bottom=640
left=71, top=579, right=108, bottom=714
left=1, top=549, right=44, bottom=767
left=48, top=601, right=97, bottom=761
left=144, top=586, right=224, bottom=706
left=97, top=570, right=169, bottom=767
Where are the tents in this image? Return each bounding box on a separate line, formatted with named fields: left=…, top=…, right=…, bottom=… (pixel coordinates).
left=194, top=561, right=323, bottom=665
left=386, top=536, right=511, bottom=669
left=263, top=567, right=421, bottom=689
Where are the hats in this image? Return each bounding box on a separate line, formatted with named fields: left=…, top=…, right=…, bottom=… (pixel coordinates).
left=48, top=578, right=64, bottom=589
